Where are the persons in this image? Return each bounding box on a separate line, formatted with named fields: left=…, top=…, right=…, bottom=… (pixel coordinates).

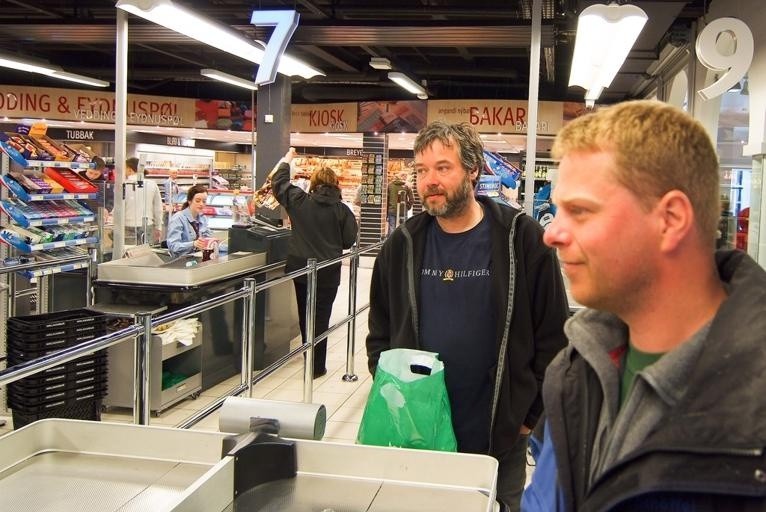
left=354, top=185, right=362, bottom=207
left=123, top=158, right=163, bottom=245
left=166, top=184, right=221, bottom=259
left=386, top=174, right=415, bottom=240
left=714, top=194, right=740, bottom=251
left=364, top=121, right=571, bottom=512
left=77, top=154, right=113, bottom=224
left=518, top=99, right=766, bottom=512
left=162, top=169, right=181, bottom=206
left=269, top=151, right=358, bottom=380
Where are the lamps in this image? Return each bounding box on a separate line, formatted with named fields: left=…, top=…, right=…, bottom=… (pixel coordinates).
left=0, top=52, right=111, bottom=89
left=114, top=1, right=327, bottom=89
left=387, top=72, right=425, bottom=94
left=567, top=1, right=649, bottom=109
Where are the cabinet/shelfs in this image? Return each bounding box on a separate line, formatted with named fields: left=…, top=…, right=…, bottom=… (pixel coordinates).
left=0, top=132, right=101, bottom=278
left=142, top=169, right=253, bottom=205
left=518, top=151, right=560, bottom=198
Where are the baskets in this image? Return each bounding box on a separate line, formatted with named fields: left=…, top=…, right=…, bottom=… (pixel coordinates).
left=7, top=309, right=108, bottom=430
